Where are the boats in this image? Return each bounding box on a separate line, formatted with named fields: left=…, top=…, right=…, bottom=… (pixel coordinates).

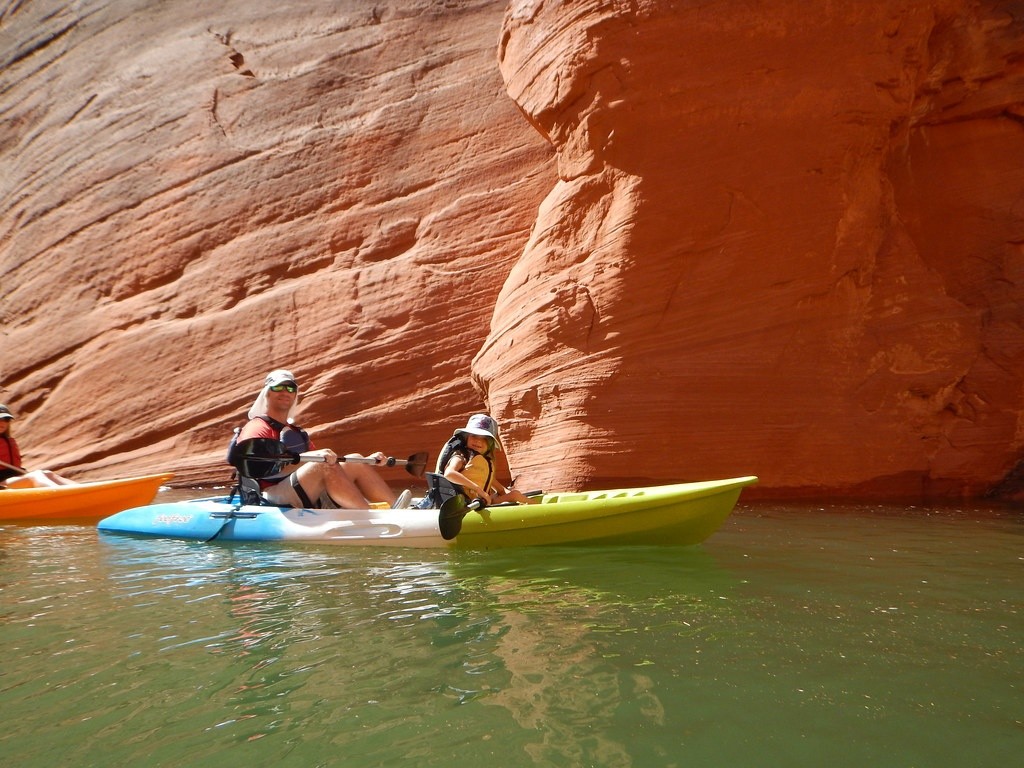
left=95, top=476, right=759, bottom=557
left=1, top=473, right=173, bottom=523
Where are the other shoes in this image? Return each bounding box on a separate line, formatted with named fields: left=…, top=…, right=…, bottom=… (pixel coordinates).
left=391, top=488, right=413, bottom=509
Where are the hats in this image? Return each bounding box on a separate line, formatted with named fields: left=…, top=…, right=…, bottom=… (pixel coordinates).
left=248, top=369, right=298, bottom=423
left=453, top=414, right=502, bottom=450
left=0, top=405, right=14, bottom=420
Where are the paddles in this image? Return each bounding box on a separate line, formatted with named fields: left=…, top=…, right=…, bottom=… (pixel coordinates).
left=230, top=436, right=431, bottom=480
left=436, top=488, right=546, bottom=541
left=0, top=460, right=29, bottom=474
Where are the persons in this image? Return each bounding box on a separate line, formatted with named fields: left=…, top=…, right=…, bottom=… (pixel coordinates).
left=236, top=370, right=413, bottom=509
left=435, top=414, right=535, bottom=506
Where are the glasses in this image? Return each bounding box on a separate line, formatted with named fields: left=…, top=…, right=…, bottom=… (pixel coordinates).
left=3, top=417, right=11, bottom=422
left=270, top=385, right=295, bottom=393
